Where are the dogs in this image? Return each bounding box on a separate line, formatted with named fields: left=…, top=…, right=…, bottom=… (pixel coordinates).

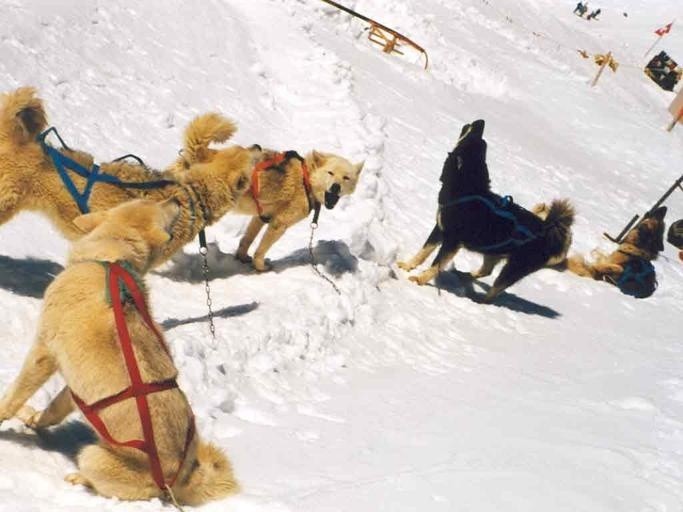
left=0, top=86, right=263, bottom=275
left=564, top=206, right=668, bottom=298
left=2, top=196, right=241, bottom=507
left=169, top=112, right=365, bottom=273
left=529, top=201, right=573, bottom=266
left=396, top=119, right=576, bottom=305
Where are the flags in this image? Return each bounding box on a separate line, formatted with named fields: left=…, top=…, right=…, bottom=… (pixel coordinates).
left=654, top=22, right=672, bottom=36
